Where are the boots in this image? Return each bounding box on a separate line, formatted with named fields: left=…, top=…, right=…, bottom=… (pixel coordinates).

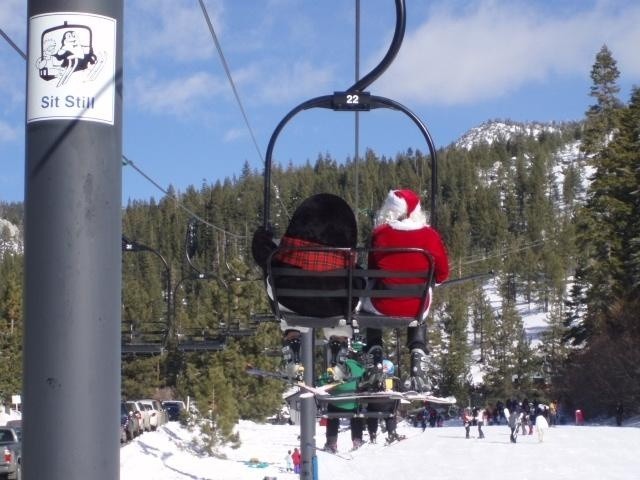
left=326, top=335, right=352, bottom=383
left=358, top=344, right=384, bottom=391
left=385, top=188, right=422, bottom=221
left=278, top=332, right=304, bottom=381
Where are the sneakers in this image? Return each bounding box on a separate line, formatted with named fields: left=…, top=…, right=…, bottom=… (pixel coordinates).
left=410, top=348, right=435, bottom=393
left=388, top=430, right=406, bottom=439
left=352, top=437, right=365, bottom=448
left=370, top=432, right=377, bottom=444
left=324, top=435, right=337, bottom=452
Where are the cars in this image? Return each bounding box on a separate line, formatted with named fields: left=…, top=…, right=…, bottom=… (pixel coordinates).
left=1, top=426, right=22, bottom=480
left=120, top=399, right=185, bottom=443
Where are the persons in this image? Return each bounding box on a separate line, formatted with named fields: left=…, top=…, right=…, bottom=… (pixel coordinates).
left=615, top=401, right=623, bottom=426
left=291, top=448, right=300, bottom=474
left=361, top=188, right=450, bottom=390
left=318, top=342, right=558, bottom=455
left=284, top=450, right=293, bottom=473
left=251, top=190, right=367, bottom=381
